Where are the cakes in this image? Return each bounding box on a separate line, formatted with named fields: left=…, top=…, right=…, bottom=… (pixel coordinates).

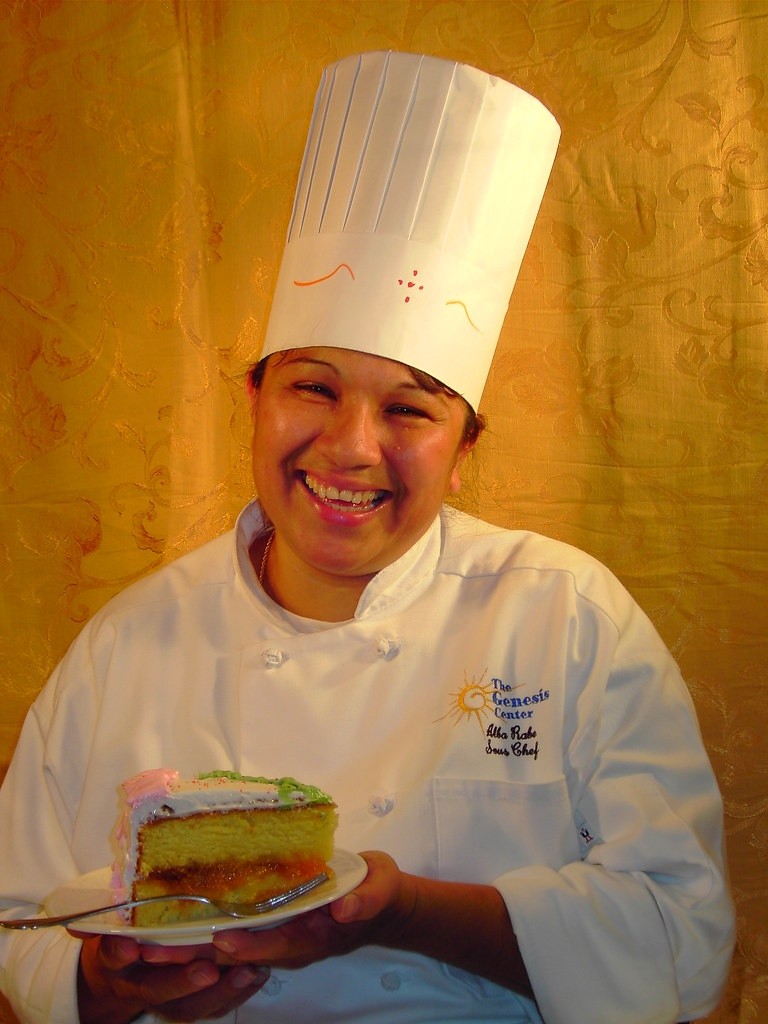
left=107, top=767, right=339, bottom=927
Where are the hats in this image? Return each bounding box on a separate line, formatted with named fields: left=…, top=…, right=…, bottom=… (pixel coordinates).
left=259, top=52, right=558, bottom=414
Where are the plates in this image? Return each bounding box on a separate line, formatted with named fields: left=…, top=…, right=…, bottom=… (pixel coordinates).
left=41, top=847, right=368, bottom=946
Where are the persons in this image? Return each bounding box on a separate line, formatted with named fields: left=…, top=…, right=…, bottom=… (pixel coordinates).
left=0, top=51, right=735, bottom=1024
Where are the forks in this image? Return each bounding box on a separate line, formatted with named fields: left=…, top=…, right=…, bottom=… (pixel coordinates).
left=0, top=872, right=329, bottom=930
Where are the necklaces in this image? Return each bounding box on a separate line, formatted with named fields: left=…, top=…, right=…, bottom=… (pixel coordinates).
left=260, top=530, right=274, bottom=583
